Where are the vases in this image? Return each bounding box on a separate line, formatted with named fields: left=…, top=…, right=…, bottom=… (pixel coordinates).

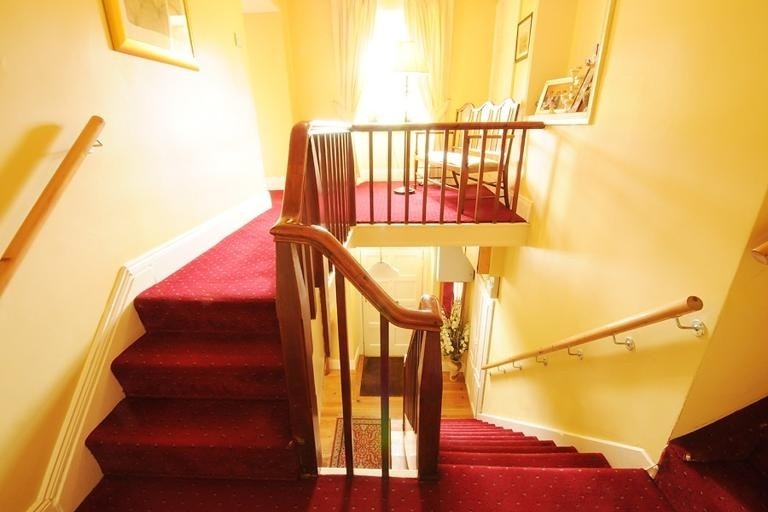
left=444, top=357, right=462, bottom=383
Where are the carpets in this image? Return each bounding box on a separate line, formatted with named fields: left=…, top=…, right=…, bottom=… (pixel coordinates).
left=329, top=357, right=406, bottom=471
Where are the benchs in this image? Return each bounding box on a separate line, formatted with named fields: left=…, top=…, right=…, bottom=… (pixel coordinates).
left=413, top=96, right=521, bottom=214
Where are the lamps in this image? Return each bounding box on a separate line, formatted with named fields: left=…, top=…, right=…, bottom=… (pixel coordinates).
left=364, top=249, right=412, bottom=286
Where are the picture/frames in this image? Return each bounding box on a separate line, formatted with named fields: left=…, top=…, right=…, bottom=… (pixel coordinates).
left=514, top=12, right=533, bottom=62
left=102, top=0, right=203, bottom=72
left=535, top=77, right=580, bottom=115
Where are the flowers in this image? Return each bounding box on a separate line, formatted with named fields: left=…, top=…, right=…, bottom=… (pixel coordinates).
left=434, top=294, right=471, bottom=360
